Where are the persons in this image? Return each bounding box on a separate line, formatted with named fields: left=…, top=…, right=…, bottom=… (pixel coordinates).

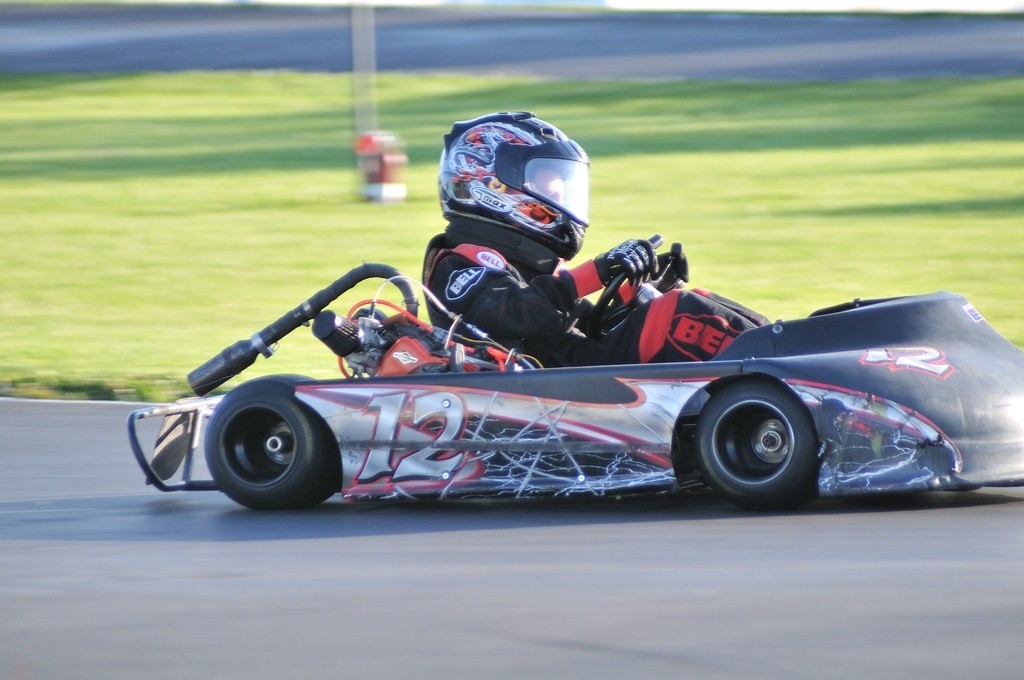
left=423, top=111, right=771, bottom=369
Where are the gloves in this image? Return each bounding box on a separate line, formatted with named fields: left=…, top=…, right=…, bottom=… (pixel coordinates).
left=647, top=251, right=691, bottom=295
left=594, top=239, right=660, bottom=287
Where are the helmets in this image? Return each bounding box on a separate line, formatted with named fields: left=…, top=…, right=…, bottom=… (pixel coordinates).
left=438, top=111, right=591, bottom=261
